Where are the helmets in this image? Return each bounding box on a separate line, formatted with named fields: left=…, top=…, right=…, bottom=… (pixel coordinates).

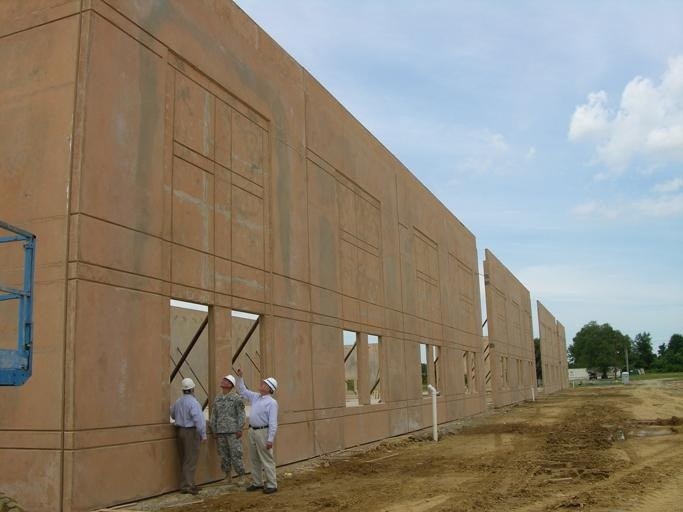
left=224, top=375, right=236, bottom=387
left=264, top=376, right=278, bottom=392
left=181, top=378, right=195, bottom=391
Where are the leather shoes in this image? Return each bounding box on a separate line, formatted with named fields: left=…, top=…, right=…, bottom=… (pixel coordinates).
left=180, top=489, right=199, bottom=494
left=262, top=488, right=278, bottom=493
left=247, top=485, right=264, bottom=490
left=191, top=486, right=202, bottom=491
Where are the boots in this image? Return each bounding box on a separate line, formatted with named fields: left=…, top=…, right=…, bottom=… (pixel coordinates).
left=237, top=472, right=246, bottom=486
left=221, top=472, right=232, bottom=483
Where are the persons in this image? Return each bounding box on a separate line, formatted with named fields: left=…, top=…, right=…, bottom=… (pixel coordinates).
left=171, top=378, right=207, bottom=495
left=209, top=375, right=246, bottom=487
left=236, top=368, right=279, bottom=495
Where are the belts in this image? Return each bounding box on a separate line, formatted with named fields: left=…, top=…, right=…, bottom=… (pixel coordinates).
left=249, top=425, right=268, bottom=430
left=187, top=427, right=196, bottom=429
